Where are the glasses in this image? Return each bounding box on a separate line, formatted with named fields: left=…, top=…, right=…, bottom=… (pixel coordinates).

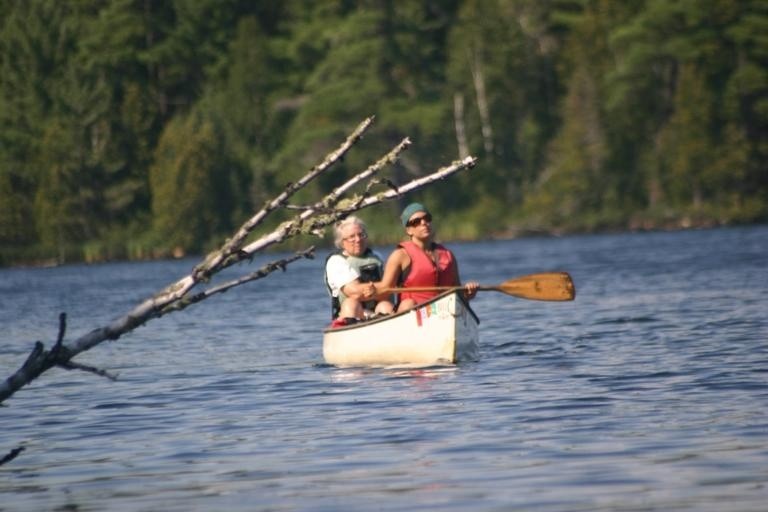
left=405, top=213, right=432, bottom=226
left=341, top=232, right=368, bottom=242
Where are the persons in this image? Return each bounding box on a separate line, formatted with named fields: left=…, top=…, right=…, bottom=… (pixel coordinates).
left=362, top=202, right=480, bottom=316
left=323, top=216, right=395, bottom=325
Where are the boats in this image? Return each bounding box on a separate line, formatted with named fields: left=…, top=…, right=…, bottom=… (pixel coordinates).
left=321, top=288, right=482, bottom=370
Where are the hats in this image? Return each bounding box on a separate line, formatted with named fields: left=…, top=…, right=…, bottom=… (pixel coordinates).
left=401, top=202, right=429, bottom=228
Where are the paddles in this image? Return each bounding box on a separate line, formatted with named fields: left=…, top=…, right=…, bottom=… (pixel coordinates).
left=371, top=272, right=575, bottom=301
